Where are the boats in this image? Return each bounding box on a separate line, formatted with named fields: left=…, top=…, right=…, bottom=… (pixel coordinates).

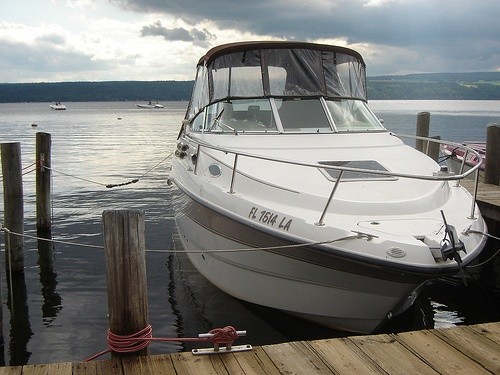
left=170, top=40, right=489, bottom=334
left=49, top=104, right=66, bottom=110
left=137, top=104, right=164, bottom=108
